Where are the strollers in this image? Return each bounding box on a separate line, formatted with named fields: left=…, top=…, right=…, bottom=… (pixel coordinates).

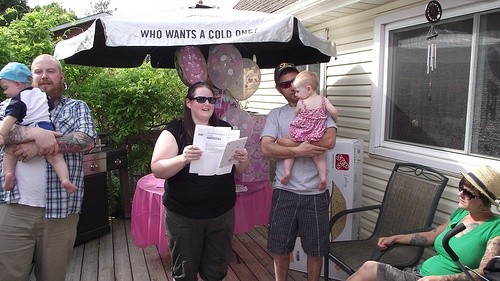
left=442, top=222, right=500, bottom=281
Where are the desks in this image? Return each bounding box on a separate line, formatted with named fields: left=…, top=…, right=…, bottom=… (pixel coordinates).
left=133, top=174, right=271, bottom=264
left=74, top=147, right=128, bottom=247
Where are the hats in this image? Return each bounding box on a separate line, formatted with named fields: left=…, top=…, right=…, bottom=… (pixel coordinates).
left=460, top=164, right=500, bottom=207
left=274, top=62, right=299, bottom=82
left=0, top=62, right=32, bottom=83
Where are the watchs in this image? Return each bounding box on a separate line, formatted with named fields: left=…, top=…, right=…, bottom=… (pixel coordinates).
left=274, top=137, right=278, bottom=145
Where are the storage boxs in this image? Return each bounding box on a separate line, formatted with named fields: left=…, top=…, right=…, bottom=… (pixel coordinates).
left=287, top=137, right=364, bottom=281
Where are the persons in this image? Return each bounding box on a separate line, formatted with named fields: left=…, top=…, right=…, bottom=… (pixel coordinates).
left=0, top=54, right=96, bottom=281
left=346, top=165, right=500, bottom=281
left=0, top=62, right=78, bottom=192
left=259, top=62, right=337, bottom=281
left=151, top=81, right=250, bottom=281
left=280, top=70, right=338, bottom=192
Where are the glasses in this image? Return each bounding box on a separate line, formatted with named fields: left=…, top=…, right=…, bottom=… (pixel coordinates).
left=188, top=96, right=216, bottom=104
left=277, top=78, right=294, bottom=89
left=459, top=185, right=480, bottom=199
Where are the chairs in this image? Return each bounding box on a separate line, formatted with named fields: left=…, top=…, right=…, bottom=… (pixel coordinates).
left=324, top=162, right=500, bottom=281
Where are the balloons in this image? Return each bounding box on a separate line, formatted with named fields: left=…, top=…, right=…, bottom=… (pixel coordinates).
left=207, top=44, right=243, bottom=90
left=227, top=58, right=261, bottom=101
left=220, top=108, right=254, bottom=147
left=174, top=46, right=207, bottom=87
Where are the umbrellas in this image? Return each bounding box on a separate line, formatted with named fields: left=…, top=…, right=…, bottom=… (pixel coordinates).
left=52, top=0, right=336, bottom=69
